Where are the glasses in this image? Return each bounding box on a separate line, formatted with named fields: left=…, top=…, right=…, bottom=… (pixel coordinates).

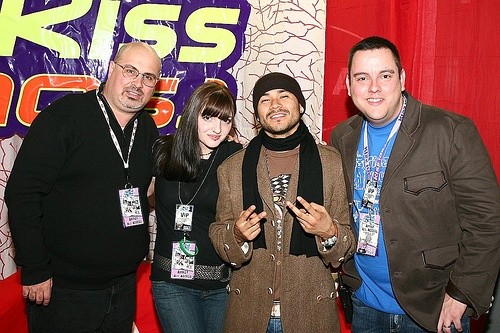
left=114, top=61, right=159, bottom=87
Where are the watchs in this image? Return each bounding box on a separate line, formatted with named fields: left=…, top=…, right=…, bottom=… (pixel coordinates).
left=318, top=221, right=338, bottom=250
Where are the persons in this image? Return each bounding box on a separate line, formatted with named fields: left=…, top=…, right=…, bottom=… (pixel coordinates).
left=147, top=77, right=244, bottom=333
left=207, top=71, right=357, bottom=333
left=328, top=35, right=500, bottom=333
left=3, top=42, right=245, bottom=333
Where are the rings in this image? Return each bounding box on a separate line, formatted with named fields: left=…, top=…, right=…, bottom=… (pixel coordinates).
left=443, top=325, right=451, bottom=328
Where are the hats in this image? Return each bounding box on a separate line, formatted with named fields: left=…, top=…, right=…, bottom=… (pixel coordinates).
left=253, top=72, right=306, bottom=118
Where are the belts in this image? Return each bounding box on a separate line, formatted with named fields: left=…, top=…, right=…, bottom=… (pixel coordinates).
left=152, top=254, right=232, bottom=281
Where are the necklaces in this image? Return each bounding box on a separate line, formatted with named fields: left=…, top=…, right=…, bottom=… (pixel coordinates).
left=178, top=147, right=220, bottom=206
